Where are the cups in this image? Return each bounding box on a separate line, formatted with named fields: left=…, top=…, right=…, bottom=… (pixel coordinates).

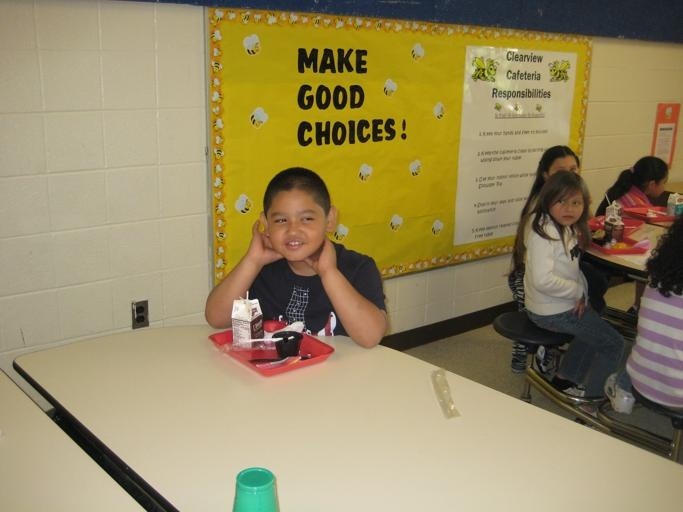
left=234, top=467, right=281, bottom=512
left=273, top=331, right=303, bottom=358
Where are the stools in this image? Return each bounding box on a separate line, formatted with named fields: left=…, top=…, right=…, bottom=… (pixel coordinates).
left=493, top=311, right=573, bottom=401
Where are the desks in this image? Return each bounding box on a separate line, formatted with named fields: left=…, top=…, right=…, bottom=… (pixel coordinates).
left=0, top=320, right=683, bottom=511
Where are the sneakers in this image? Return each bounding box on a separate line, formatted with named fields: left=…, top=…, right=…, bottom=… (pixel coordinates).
left=551, top=375, right=585, bottom=398
left=532, top=345, right=554, bottom=375
left=603, top=373, right=635, bottom=415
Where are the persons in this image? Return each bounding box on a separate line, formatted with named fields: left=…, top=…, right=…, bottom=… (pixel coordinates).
left=508, top=146, right=580, bottom=378
left=522, top=170, right=626, bottom=425
left=204, top=167, right=389, bottom=348
left=580, top=156, right=683, bottom=316
left=625, top=209, right=683, bottom=432
